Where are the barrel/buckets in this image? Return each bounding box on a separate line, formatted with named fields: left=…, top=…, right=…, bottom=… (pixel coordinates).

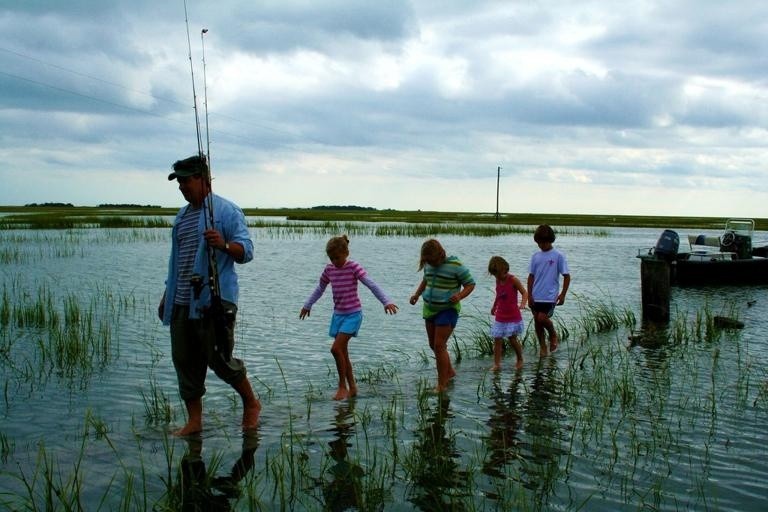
left=654, top=230, right=679, bottom=255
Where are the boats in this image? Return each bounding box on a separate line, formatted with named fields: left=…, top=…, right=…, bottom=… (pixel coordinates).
left=635, top=217, right=768, bottom=289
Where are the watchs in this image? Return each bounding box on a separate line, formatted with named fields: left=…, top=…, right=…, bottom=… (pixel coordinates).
left=223, top=238, right=230, bottom=253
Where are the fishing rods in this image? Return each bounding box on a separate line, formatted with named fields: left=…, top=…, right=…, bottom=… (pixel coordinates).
left=183, top=0, right=223, bottom=322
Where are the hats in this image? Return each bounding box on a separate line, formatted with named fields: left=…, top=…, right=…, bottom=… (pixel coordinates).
left=167, top=154, right=209, bottom=182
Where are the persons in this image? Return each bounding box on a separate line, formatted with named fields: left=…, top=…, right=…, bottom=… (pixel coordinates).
left=526, top=224, right=572, bottom=358
left=156, top=153, right=263, bottom=438
left=522, top=359, right=563, bottom=492
left=297, top=234, right=400, bottom=401
left=409, top=238, right=477, bottom=394
left=405, top=396, right=472, bottom=512
left=170, top=436, right=260, bottom=512
left=324, top=403, right=368, bottom=511
left=486, top=373, right=527, bottom=482
left=487, top=255, right=529, bottom=372
